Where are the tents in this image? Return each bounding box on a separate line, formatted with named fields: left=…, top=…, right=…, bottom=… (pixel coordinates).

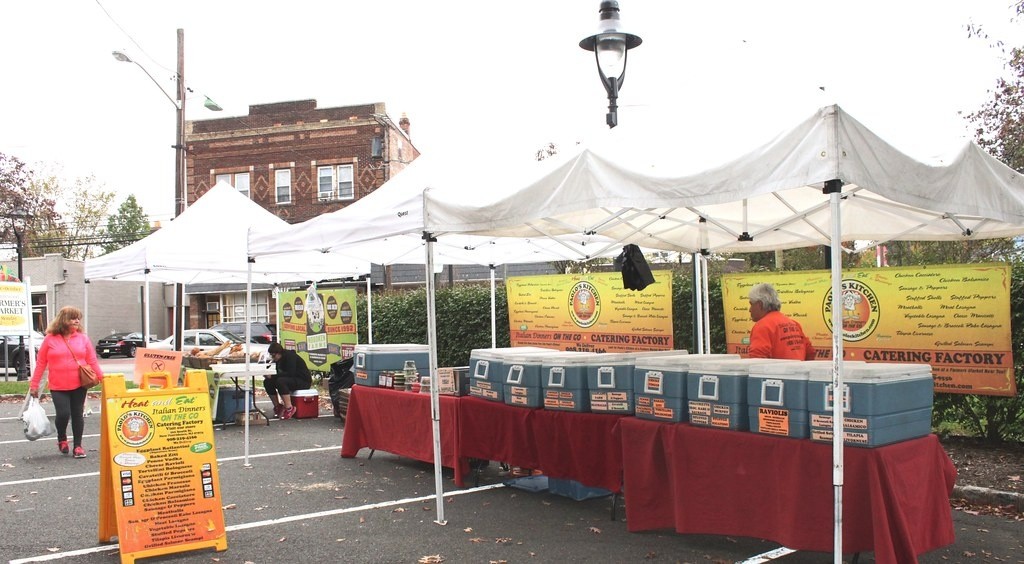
left=83, top=179, right=373, bottom=466
left=245, top=149, right=704, bottom=527
left=432, top=83, right=1024, bottom=563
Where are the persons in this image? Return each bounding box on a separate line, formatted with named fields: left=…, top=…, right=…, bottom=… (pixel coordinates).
left=263, top=343, right=312, bottom=420
left=742, top=283, right=815, bottom=360
left=28, top=306, right=104, bottom=458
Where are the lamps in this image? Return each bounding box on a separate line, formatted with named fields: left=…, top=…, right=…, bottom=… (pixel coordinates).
left=577, top=0, right=643, bottom=132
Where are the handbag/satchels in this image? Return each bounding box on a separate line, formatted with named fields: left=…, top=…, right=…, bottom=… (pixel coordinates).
left=22, top=396, right=56, bottom=441
left=79, top=364, right=100, bottom=388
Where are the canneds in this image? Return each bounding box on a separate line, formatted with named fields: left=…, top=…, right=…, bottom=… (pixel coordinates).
left=379, top=370, right=396, bottom=389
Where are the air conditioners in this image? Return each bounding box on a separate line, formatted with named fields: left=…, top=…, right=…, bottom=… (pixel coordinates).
left=207, top=302, right=219, bottom=311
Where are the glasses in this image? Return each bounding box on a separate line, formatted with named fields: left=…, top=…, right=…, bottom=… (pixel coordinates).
left=271, top=352, right=279, bottom=358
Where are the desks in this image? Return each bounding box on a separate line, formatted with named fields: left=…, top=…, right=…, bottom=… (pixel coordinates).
left=180, top=365, right=277, bottom=429
left=342, top=384, right=958, bottom=564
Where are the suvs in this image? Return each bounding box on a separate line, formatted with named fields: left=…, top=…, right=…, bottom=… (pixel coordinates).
left=208, top=322, right=277, bottom=344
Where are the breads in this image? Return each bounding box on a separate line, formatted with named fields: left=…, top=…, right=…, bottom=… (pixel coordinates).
left=186, top=340, right=261, bottom=358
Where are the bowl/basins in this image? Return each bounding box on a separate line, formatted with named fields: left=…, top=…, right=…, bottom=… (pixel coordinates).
left=403, top=360, right=421, bottom=393
left=419, top=377, right=431, bottom=394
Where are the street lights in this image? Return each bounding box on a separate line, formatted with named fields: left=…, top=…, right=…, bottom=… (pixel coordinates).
left=110, top=27, right=221, bottom=353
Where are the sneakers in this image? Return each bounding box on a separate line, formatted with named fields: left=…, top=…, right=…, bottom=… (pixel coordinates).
left=273, top=404, right=284, bottom=418
left=73, top=447, right=86, bottom=458
left=280, top=405, right=296, bottom=419
left=59, top=440, right=69, bottom=453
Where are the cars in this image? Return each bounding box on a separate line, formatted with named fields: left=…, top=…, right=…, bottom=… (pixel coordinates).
left=146, top=329, right=247, bottom=357
left=215, top=330, right=258, bottom=344
left=0, top=329, right=47, bottom=376
left=95, top=332, right=164, bottom=358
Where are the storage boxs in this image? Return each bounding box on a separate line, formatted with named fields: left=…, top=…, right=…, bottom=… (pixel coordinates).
left=546, top=476, right=610, bottom=501
left=468, top=345, right=559, bottom=401
left=503, top=351, right=596, bottom=406
left=634, top=354, right=741, bottom=422
left=542, top=352, right=621, bottom=411
left=586, top=350, right=688, bottom=413
left=236, top=410, right=267, bottom=425
left=748, top=361, right=866, bottom=438
left=352, top=343, right=429, bottom=386
left=277, top=389, right=319, bottom=418
left=436, top=366, right=470, bottom=395
left=216, top=383, right=252, bottom=422
left=687, top=358, right=800, bottom=430
left=807, top=364, right=935, bottom=447
